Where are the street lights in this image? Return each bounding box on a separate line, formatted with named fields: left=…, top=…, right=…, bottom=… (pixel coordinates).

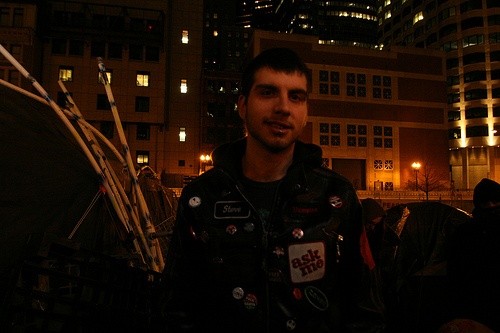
left=412, top=162, right=420, bottom=191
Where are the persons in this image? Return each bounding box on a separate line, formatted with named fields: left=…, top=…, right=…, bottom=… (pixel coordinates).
left=361, top=179, right=500, bottom=333
left=149, top=47, right=385, bottom=333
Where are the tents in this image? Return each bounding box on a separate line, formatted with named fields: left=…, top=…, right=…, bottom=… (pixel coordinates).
left=0, top=85, right=166, bottom=333
